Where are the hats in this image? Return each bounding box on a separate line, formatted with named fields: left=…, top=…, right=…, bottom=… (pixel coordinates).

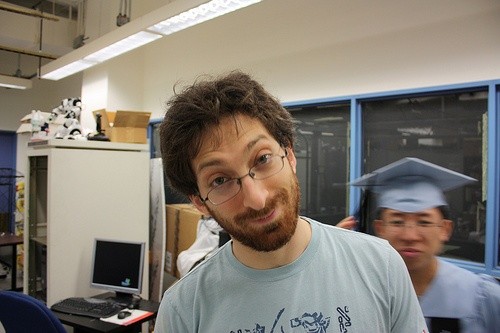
left=351, top=156, right=478, bottom=213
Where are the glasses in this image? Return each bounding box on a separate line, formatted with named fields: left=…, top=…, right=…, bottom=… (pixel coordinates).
left=381, top=218, right=446, bottom=233
left=199, top=149, right=288, bottom=206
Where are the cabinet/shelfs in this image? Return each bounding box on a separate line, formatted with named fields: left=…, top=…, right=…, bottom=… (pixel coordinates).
left=23, top=149, right=149, bottom=333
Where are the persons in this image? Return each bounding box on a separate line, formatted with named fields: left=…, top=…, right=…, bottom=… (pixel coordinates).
left=150, top=73, right=428, bottom=333
left=336, top=158, right=500, bottom=333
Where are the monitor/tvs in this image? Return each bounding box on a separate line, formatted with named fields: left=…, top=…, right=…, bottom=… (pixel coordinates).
left=90, top=239, right=145, bottom=306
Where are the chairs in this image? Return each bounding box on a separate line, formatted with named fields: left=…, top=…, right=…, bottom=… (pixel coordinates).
left=0, top=290, right=67, bottom=333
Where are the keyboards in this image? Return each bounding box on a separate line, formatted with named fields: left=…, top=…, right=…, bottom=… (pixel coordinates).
left=50, top=298, right=121, bottom=318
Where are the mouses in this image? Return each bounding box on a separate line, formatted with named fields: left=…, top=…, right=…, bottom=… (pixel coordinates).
left=118, top=311, right=131, bottom=319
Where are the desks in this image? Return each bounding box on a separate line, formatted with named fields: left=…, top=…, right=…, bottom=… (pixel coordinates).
left=0, top=234, right=24, bottom=291
left=50, top=293, right=159, bottom=333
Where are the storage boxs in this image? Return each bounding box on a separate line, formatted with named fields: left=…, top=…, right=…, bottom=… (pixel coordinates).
left=164, top=204, right=213, bottom=279
left=93, top=108, right=151, bottom=144
